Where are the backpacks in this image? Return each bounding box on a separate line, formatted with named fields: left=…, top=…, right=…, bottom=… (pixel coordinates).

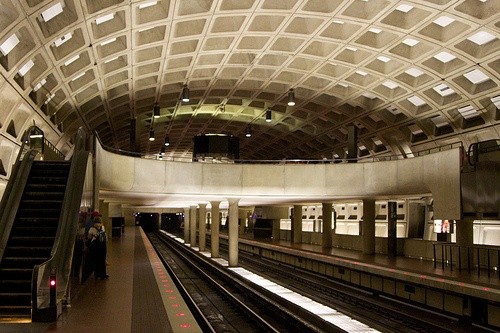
left=92, top=227, right=106, bottom=242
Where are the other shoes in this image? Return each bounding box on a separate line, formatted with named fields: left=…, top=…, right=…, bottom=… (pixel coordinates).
left=95, top=277, right=101, bottom=280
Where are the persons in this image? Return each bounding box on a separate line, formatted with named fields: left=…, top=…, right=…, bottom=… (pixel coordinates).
left=87, top=215, right=109, bottom=279
left=85, top=210, right=102, bottom=230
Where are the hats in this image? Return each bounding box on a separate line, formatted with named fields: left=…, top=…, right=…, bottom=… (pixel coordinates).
left=91, top=211, right=101, bottom=220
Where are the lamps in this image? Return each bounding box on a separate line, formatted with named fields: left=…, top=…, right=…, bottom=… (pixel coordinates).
left=183, top=84, right=189, bottom=102
left=288, top=88, right=294, bottom=107
left=150, top=128, right=155, bottom=141
left=159, top=134, right=171, bottom=160
left=265, top=107, right=272, bottom=123
left=153, top=102, right=161, bottom=119
left=246, top=126, right=253, bottom=138
